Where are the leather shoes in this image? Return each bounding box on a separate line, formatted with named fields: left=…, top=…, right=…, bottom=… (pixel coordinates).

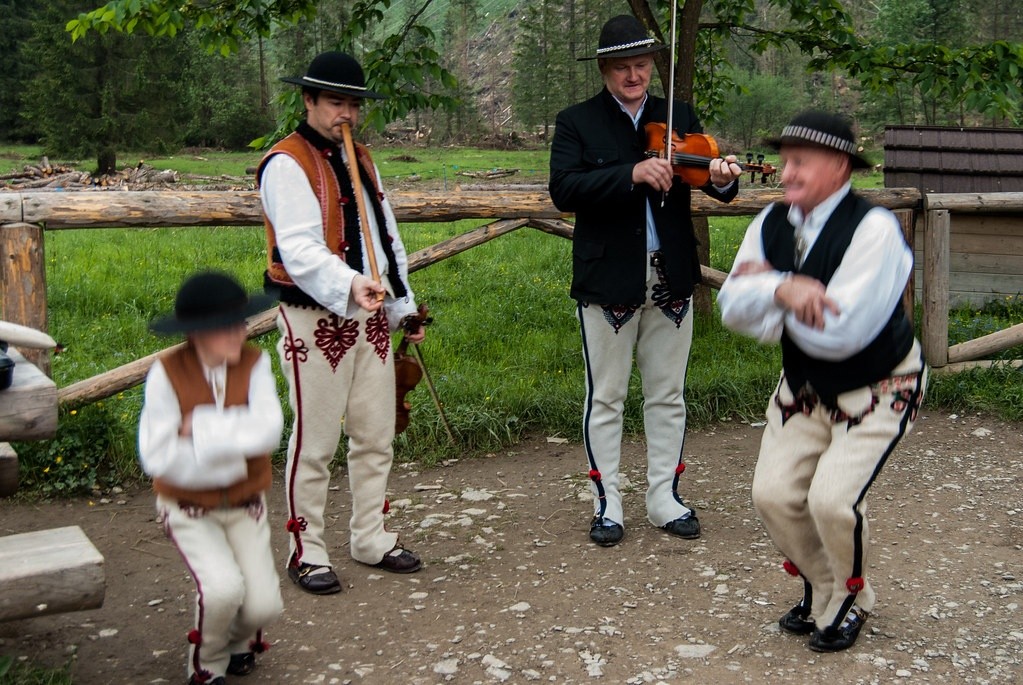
left=288, top=561, right=342, bottom=594
left=808, top=605, right=870, bottom=652
left=227, top=654, right=256, bottom=675
left=778, top=597, right=815, bottom=635
left=374, top=544, right=422, bottom=573
left=589, top=522, right=624, bottom=546
left=660, top=514, right=700, bottom=538
left=190, top=677, right=225, bottom=685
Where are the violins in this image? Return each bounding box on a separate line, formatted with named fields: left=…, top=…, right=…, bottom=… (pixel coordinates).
left=642, top=119, right=776, bottom=188
left=393, top=302, right=428, bottom=434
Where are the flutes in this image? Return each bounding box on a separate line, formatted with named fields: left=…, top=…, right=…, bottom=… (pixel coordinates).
left=341, top=120, right=384, bottom=303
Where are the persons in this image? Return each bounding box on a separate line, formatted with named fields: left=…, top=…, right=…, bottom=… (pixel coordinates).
left=716, top=107, right=935, bottom=655
left=254, top=52, right=430, bottom=595
left=138, top=274, right=290, bottom=685
left=549, top=13, right=739, bottom=549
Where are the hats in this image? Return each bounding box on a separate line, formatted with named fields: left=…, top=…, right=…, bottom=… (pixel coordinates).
left=763, top=108, right=873, bottom=170
left=575, top=13, right=672, bottom=61
left=277, top=51, right=390, bottom=99
left=148, top=271, right=276, bottom=336
